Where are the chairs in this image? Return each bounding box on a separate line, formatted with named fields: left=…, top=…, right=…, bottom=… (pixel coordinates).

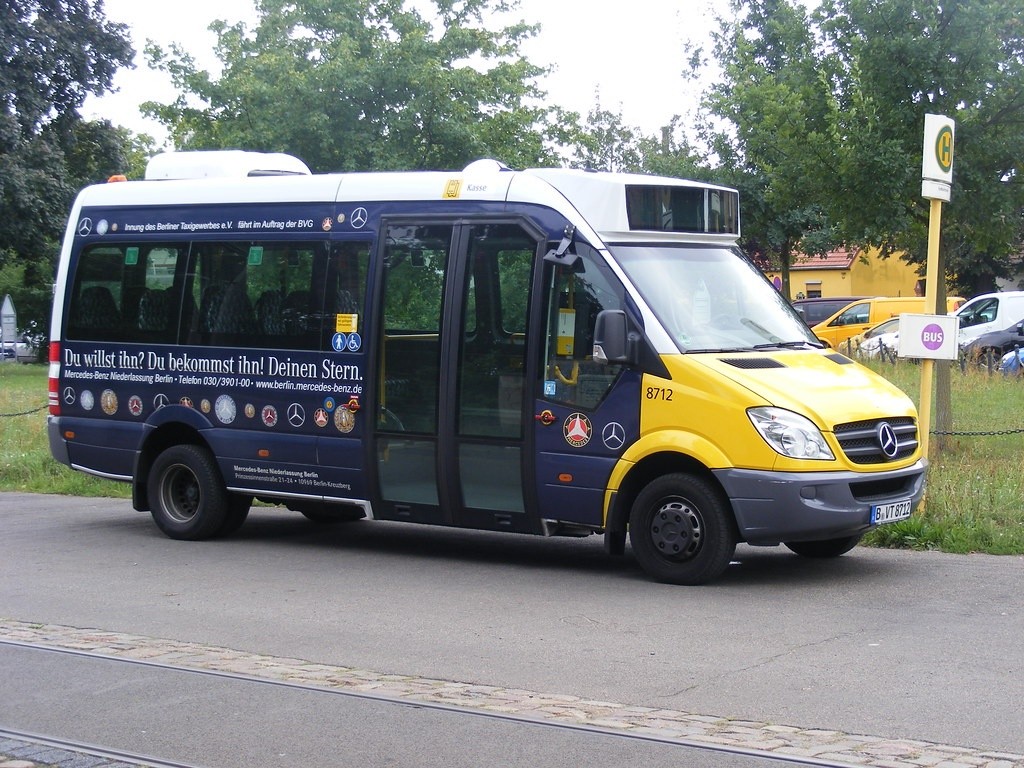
left=76, top=281, right=347, bottom=355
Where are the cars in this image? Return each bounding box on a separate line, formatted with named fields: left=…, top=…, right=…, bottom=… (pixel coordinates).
left=962, top=320, right=1024, bottom=363
left=0, top=330, right=46, bottom=364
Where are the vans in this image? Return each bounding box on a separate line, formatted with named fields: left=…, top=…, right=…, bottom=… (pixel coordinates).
left=811, top=289, right=1024, bottom=352
left=44, top=147, right=931, bottom=588
left=788, top=293, right=872, bottom=331
